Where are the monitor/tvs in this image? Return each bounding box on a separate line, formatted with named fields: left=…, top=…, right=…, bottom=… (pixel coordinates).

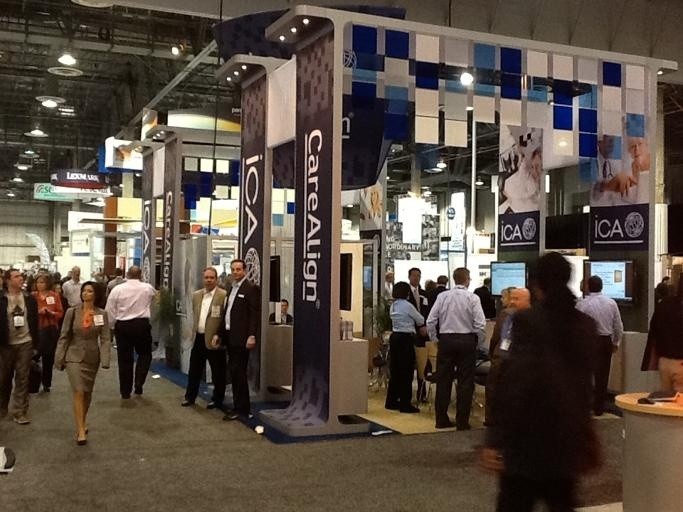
left=363, top=263, right=373, bottom=291
left=269, top=255, right=280, bottom=302
left=490, top=261, right=527, bottom=297
left=583, top=259, right=637, bottom=307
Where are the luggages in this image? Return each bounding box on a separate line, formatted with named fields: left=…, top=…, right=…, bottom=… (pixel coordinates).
left=27, top=347, right=42, bottom=393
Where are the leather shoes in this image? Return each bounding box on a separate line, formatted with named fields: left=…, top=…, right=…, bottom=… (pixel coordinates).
left=228, top=410, right=240, bottom=420
left=207, top=401, right=217, bottom=409
left=76, top=431, right=88, bottom=445
left=181, top=400, right=191, bottom=406
left=400, top=405, right=420, bottom=413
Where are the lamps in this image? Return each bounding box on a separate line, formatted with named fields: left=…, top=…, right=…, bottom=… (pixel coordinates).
left=475, top=176, right=484, bottom=186
left=171, top=44, right=184, bottom=55
left=424, top=158, right=447, bottom=174
left=5, top=13, right=83, bottom=198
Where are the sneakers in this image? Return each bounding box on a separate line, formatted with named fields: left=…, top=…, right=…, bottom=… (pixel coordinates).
left=12, top=414, right=31, bottom=424
left=435, top=421, right=456, bottom=428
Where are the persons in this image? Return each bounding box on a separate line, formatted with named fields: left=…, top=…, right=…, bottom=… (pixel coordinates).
left=496, top=252, right=603, bottom=511
left=0, top=265, right=161, bottom=446
left=574, top=274, right=623, bottom=416
left=503, top=141, right=542, bottom=213
left=180, top=259, right=259, bottom=420
left=360, top=187, right=381, bottom=220
left=269, top=299, right=293, bottom=325
left=116, top=145, right=133, bottom=162
left=385, top=251, right=533, bottom=473
left=648, top=271, right=683, bottom=393
left=592, top=133, right=650, bottom=207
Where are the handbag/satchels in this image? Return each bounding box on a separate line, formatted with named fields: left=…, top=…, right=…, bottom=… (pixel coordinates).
left=641, top=298, right=663, bottom=371
